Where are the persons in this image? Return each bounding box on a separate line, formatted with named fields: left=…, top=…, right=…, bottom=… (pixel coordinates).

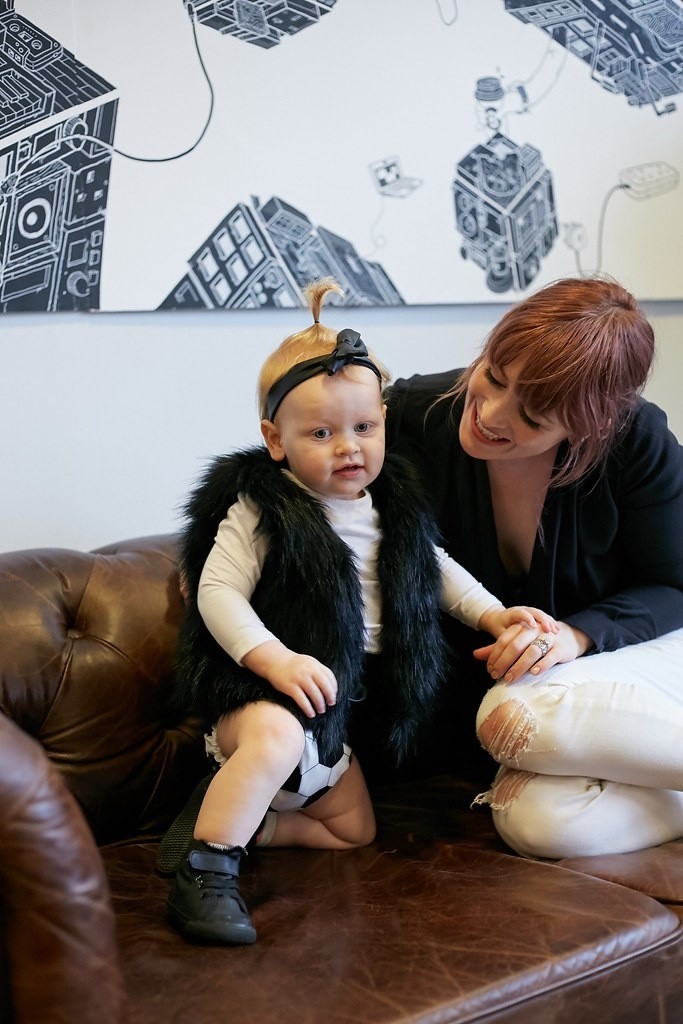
left=155, top=275, right=559, bottom=944
left=383, top=278, right=683, bottom=858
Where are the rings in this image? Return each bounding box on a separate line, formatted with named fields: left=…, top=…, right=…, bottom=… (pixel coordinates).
left=529, top=638, right=549, bottom=656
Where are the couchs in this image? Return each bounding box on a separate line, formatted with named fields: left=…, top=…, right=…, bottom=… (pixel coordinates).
left=0, top=523, right=683, bottom=1024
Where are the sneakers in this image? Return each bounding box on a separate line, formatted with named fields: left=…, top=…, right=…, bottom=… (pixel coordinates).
left=163, top=840, right=258, bottom=945
left=154, top=774, right=212, bottom=876
